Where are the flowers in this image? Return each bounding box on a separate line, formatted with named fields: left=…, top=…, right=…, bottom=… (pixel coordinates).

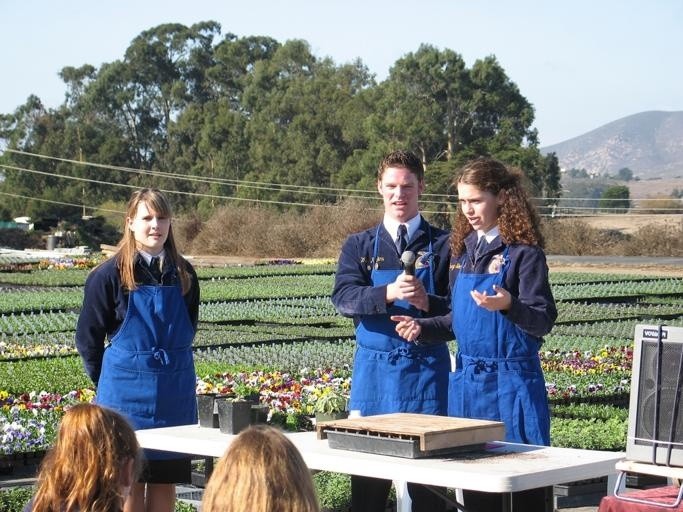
left=0, top=386, right=96, bottom=455
left=538, top=343, right=633, bottom=398
left=1, top=341, right=78, bottom=359
left=195, top=368, right=351, bottom=434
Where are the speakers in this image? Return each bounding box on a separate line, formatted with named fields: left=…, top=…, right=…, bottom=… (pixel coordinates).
left=625, top=324, right=683, bottom=468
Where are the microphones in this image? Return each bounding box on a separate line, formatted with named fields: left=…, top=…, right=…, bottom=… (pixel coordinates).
left=401, top=251, right=415, bottom=275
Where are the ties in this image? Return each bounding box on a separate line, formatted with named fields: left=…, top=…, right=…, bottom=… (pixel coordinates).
left=393, top=223, right=407, bottom=256
left=147, top=256, right=163, bottom=284
left=470, top=234, right=489, bottom=268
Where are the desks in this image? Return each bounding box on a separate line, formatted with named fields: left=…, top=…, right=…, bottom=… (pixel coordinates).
left=134, top=424, right=627, bottom=512
left=597, top=484, right=683, bottom=512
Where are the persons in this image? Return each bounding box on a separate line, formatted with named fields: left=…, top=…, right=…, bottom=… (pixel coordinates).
left=27, top=404, right=137, bottom=511
left=200, top=424, right=319, bottom=512
left=390, top=156, right=558, bottom=512
left=332, top=150, right=457, bottom=512
left=74, top=188, right=198, bottom=511
